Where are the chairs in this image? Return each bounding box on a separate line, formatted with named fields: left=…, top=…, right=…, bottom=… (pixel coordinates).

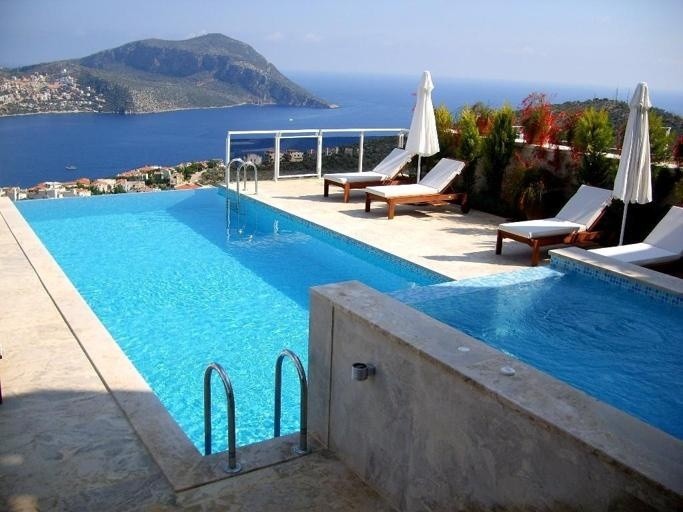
left=494, top=185, right=683, bottom=271
left=320, top=145, right=470, bottom=221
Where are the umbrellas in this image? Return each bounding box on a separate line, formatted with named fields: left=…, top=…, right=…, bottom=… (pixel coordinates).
left=405, top=70, right=441, bottom=182
left=611, top=81, right=653, bottom=246
